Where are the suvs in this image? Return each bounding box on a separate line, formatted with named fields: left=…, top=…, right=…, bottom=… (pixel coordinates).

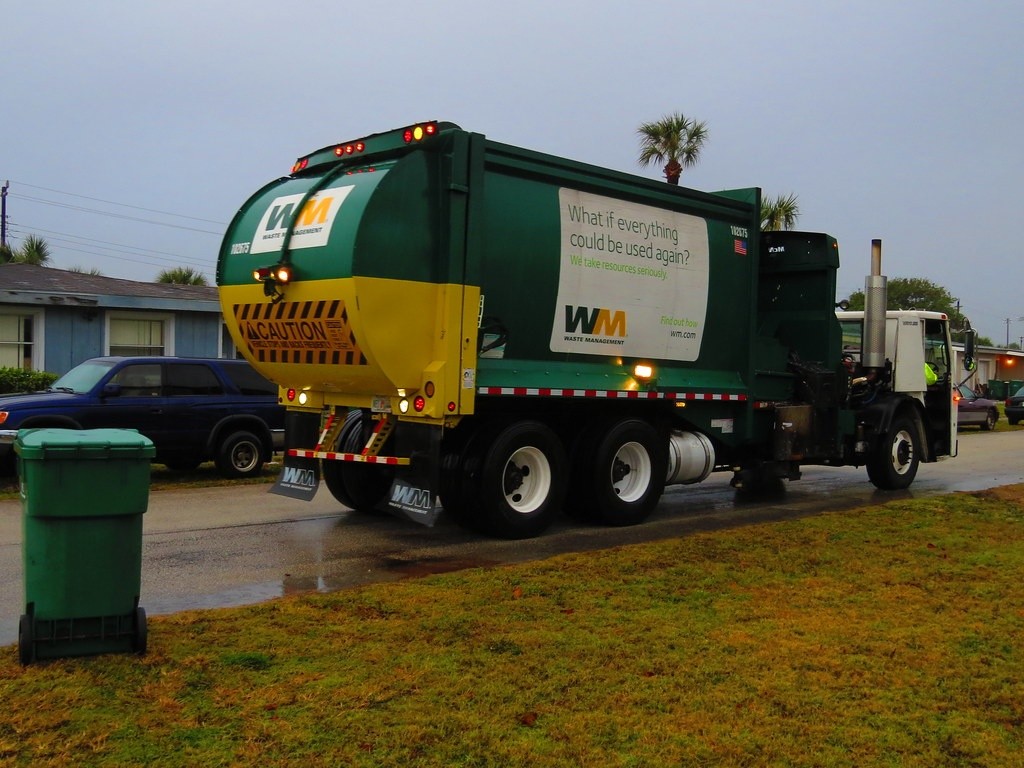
left=0, top=355, right=286, bottom=486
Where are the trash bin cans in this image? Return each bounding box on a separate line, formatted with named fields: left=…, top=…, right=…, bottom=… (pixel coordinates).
left=15, top=426, right=155, bottom=662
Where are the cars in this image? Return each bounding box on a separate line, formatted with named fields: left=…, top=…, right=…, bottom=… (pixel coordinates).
left=956, top=383, right=1000, bottom=432
left=1004, top=385, right=1024, bottom=425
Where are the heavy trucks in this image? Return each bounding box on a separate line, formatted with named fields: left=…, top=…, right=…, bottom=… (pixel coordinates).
left=217, top=120, right=976, bottom=541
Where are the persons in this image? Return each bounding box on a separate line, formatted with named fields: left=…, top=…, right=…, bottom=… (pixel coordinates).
left=925, top=361, right=940, bottom=385
